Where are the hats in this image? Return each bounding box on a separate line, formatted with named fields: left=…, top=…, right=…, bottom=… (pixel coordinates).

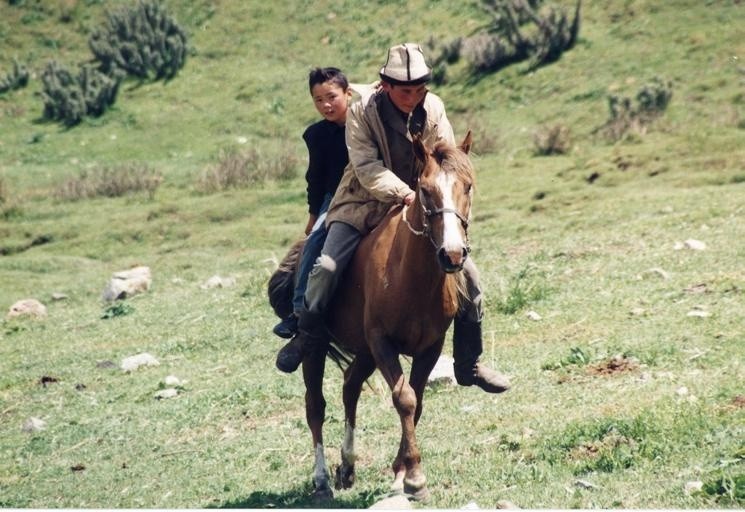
left=379, top=43, right=431, bottom=87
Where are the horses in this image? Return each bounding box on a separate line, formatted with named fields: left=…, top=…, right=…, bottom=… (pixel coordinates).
left=294, top=130, right=480, bottom=501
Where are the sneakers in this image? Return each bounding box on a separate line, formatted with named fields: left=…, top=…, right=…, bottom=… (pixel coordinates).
left=270, top=311, right=301, bottom=374
left=451, top=357, right=510, bottom=397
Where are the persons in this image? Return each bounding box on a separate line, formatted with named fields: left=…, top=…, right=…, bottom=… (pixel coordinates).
left=271, top=67, right=355, bottom=338
left=275, top=42, right=512, bottom=393
